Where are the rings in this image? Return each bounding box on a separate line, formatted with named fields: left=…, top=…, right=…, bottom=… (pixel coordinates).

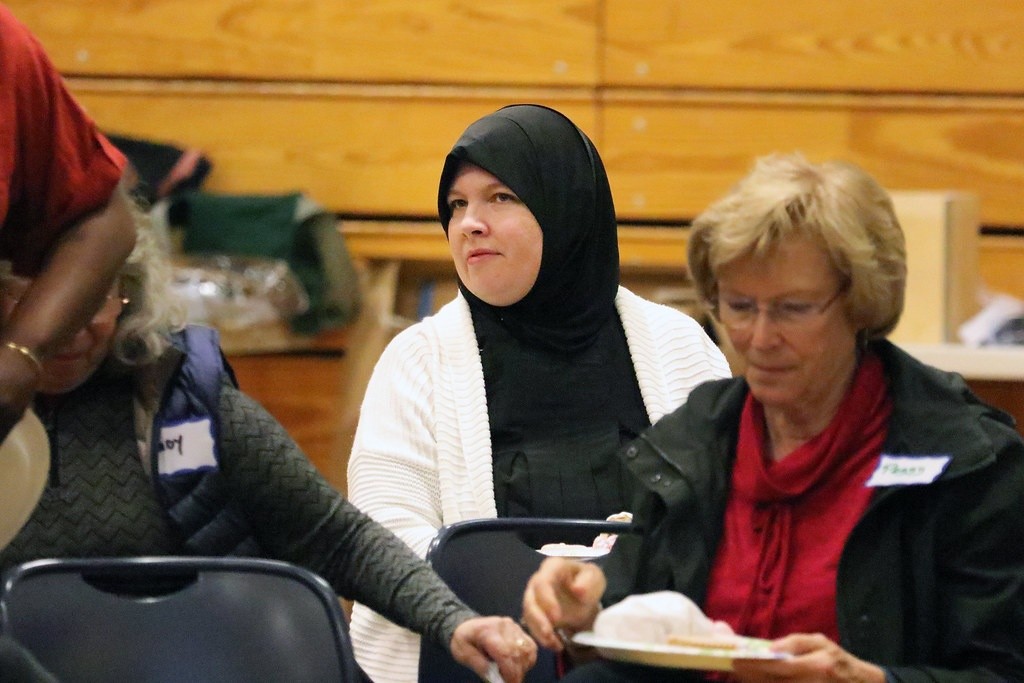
left=517, top=639, right=524, bottom=646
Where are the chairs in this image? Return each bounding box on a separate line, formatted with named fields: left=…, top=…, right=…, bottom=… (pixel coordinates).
left=418, top=517, right=642, bottom=683
left=0, top=553, right=358, bottom=683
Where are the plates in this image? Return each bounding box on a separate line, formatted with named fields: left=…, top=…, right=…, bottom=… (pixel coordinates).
left=572, top=631, right=793, bottom=671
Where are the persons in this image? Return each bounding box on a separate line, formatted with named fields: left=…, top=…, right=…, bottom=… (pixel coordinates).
left=522, top=158, right=1024, bottom=683
left=345, top=103, right=734, bottom=683
left=0, top=10, right=537, bottom=683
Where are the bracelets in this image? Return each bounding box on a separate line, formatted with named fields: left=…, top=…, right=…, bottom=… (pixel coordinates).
left=0, top=341, right=46, bottom=376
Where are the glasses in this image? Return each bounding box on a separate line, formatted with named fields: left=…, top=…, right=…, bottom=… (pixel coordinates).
left=704, top=278, right=871, bottom=328
left=94, top=295, right=129, bottom=322
